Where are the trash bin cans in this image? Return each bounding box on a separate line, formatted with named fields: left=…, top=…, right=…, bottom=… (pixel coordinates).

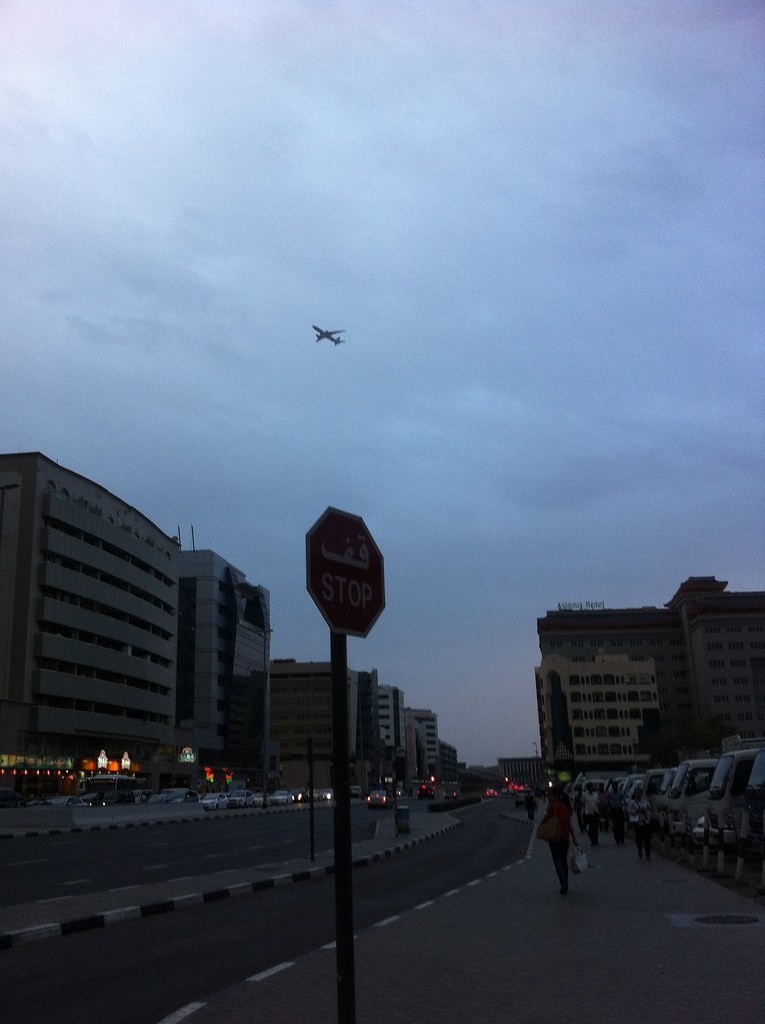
left=396, top=805, right=411, bottom=834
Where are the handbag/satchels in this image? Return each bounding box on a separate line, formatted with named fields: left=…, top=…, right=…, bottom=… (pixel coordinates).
left=537, top=804, right=561, bottom=841
left=645, top=810, right=651, bottom=824
left=570, top=846, right=589, bottom=873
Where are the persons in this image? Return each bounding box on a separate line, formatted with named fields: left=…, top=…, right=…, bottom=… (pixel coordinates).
left=605, top=781, right=628, bottom=844
left=627, top=788, right=653, bottom=860
left=574, top=786, right=587, bottom=833
left=598, top=783, right=610, bottom=834
left=525, top=791, right=534, bottom=820
left=535, top=787, right=579, bottom=895
left=581, top=782, right=601, bottom=846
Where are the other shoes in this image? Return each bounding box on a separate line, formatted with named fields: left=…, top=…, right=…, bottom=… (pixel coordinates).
left=591, top=842, right=598, bottom=845
left=561, top=886, right=568, bottom=894
left=637, top=850, right=651, bottom=861
left=616, top=840, right=625, bottom=844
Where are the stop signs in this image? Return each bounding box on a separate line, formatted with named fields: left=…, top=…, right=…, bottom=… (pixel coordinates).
left=304, top=506, right=387, bottom=638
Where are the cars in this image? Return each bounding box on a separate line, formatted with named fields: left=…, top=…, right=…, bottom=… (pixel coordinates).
left=9, top=743, right=765, bottom=887
